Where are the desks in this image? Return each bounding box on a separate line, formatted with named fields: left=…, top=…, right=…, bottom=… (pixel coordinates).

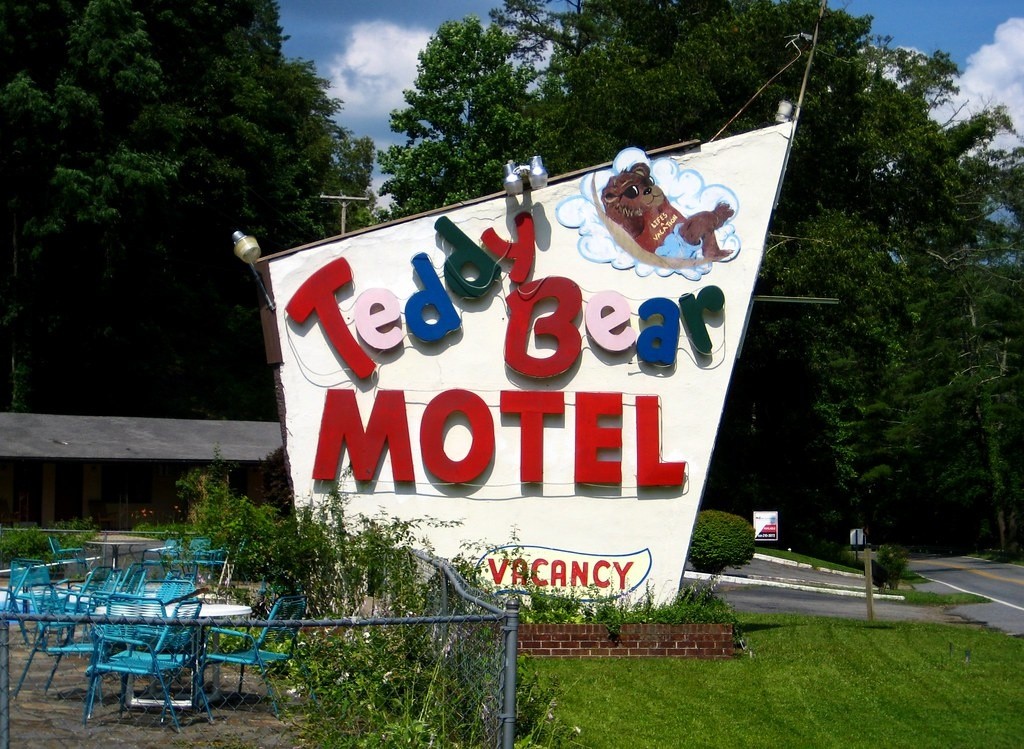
left=86, top=538, right=150, bottom=580
left=95, top=603, right=252, bottom=708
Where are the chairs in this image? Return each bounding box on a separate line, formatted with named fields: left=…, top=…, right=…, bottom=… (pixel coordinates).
left=0, top=535, right=320, bottom=734
